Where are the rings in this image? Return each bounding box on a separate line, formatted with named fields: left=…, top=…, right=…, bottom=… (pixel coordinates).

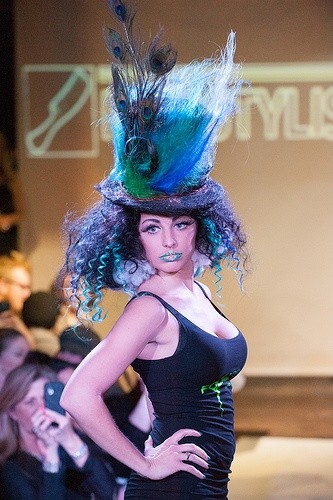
left=185, top=451, right=191, bottom=460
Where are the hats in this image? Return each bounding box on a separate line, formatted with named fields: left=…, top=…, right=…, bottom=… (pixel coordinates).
left=93, top=0, right=248, bottom=216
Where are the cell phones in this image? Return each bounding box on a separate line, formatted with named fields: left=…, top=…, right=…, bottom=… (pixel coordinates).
left=44, top=382, right=66, bottom=427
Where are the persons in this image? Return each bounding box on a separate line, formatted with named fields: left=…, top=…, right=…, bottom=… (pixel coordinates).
left=0, top=249, right=150, bottom=500
left=59, top=195, right=248, bottom=500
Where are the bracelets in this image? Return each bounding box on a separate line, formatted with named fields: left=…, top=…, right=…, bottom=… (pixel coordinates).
left=42, top=460, right=61, bottom=473
left=70, top=442, right=89, bottom=461
